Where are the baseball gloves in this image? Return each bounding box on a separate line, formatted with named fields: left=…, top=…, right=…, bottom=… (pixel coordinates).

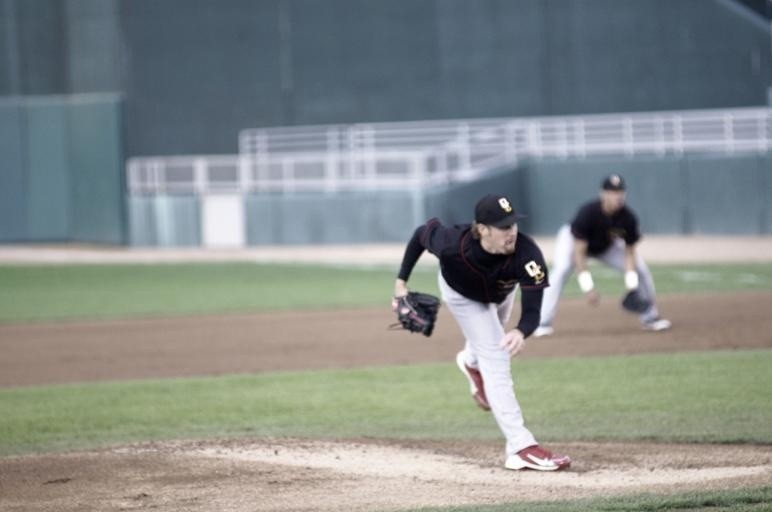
left=622, top=288, right=651, bottom=313
left=397, top=292, right=439, bottom=336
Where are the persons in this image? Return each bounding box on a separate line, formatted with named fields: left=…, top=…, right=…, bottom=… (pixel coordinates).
left=533, top=171, right=670, bottom=339
left=387, top=192, right=573, bottom=472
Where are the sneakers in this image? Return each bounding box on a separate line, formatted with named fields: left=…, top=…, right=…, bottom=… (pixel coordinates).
left=533, top=325, right=554, bottom=337
left=505, top=445, right=570, bottom=472
left=456, top=351, right=491, bottom=411
left=641, top=319, right=671, bottom=332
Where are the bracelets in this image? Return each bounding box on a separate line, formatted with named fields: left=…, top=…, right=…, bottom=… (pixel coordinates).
left=577, top=270, right=594, bottom=293
left=623, top=270, right=640, bottom=289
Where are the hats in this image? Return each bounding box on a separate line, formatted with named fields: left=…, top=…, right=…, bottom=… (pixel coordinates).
left=604, top=173, right=627, bottom=190
left=475, top=193, right=527, bottom=228
